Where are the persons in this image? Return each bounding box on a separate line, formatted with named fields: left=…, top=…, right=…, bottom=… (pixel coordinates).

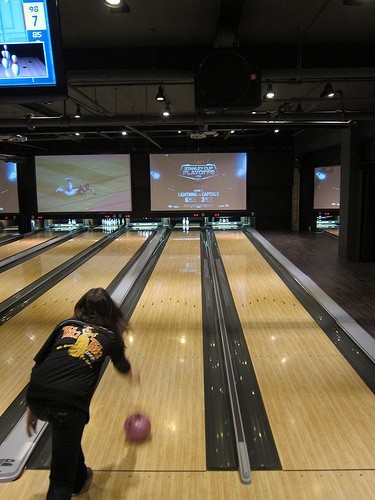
left=56, top=177, right=94, bottom=197
left=25, top=287, right=140, bottom=500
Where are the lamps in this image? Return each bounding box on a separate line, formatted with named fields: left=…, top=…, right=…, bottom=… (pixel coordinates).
left=155, top=85, right=165, bottom=102
left=322, top=82, right=336, bottom=97
left=75, top=104, right=81, bottom=118
left=265, top=83, right=275, bottom=99
left=163, top=104, right=170, bottom=116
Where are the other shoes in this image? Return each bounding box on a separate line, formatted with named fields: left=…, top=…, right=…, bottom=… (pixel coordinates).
left=70, top=467, right=92, bottom=495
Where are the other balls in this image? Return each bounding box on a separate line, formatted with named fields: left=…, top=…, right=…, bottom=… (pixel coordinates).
left=124, top=414, right=150, bottom=442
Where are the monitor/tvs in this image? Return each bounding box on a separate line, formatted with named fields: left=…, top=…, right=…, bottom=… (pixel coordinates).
left=0, top=0, right=69, bottom=103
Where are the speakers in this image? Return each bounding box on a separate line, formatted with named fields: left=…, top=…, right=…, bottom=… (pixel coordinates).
left=194, top=47, right=263, bottom=110
